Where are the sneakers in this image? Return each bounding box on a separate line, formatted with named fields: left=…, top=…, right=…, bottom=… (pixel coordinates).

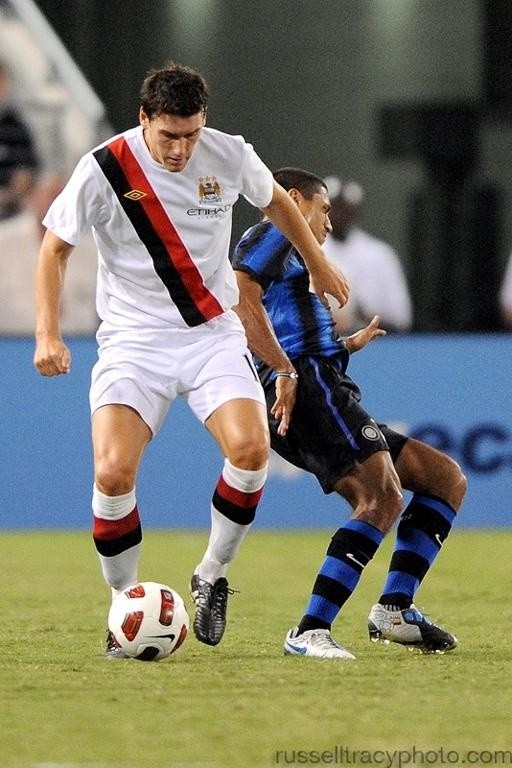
left=368, top=603, right=458, bottom=654
left=189, top=565, right=228, bottom=646
left=284, top=628, right=357, bottom=660
left=107, top=631, right=128, bottom=659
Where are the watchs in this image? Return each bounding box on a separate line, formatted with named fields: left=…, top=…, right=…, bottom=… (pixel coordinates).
left=276, top=372, right=298, bottom=378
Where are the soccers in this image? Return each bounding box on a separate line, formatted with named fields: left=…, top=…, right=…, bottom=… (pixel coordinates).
left=108, top=582, right=190, bottom=662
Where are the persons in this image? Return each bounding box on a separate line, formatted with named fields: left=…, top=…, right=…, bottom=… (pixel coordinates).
left=228, top=165, right=467, bottom=659
left=30, top=60, right=352, bottom=653
left=306, top=173, right=415, bottom=335
left=0, top=60, right=106, bottom=334
